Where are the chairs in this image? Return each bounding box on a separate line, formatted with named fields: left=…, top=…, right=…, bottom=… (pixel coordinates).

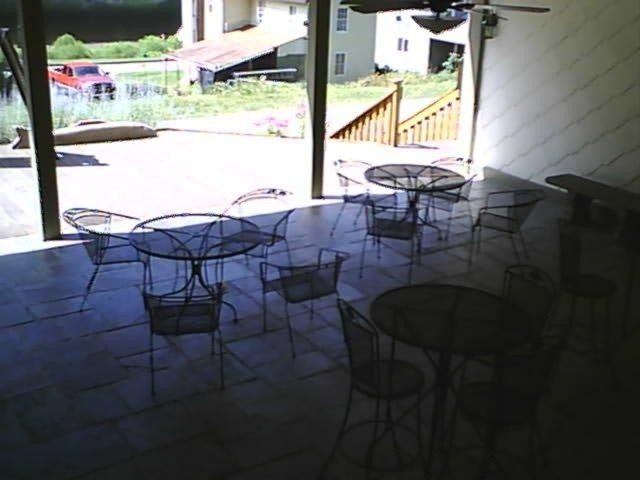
left=260, top=246, right=352, bottom=358
left=204, top=189, right=295, bottom=292
left=142, top=284, right=226, bottom=398
left=317, top=156, right=640, bottom=480
left=62, top=208, right=155, bottom=312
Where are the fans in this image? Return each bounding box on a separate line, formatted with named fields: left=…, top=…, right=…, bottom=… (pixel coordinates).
left=337, top=0, right=550, bottom=35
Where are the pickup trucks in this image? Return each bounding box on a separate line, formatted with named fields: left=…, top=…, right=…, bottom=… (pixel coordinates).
left=49, top=61, right=116, bottom=100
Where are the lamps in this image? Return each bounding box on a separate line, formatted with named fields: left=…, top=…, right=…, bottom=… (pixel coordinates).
left=480, top=12, right=509, bottom=38
left=411, top=9, right=468, bottom=37
